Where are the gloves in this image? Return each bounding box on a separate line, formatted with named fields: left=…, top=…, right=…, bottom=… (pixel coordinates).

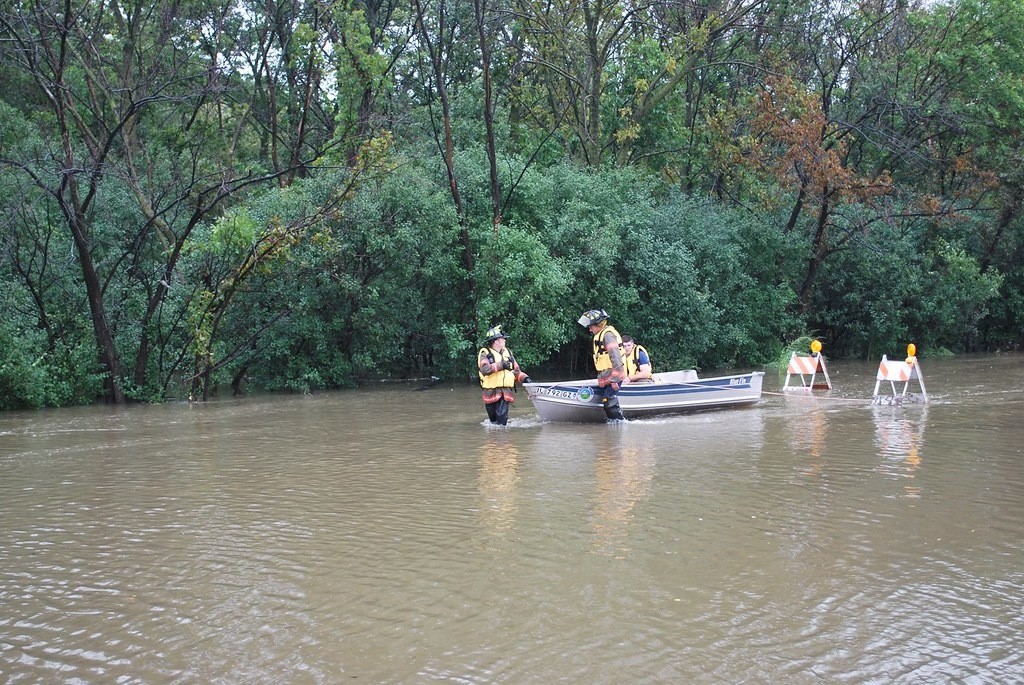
left=498, top=360, right=511, bottom=370
left=523, top=378, right=532, bottom=383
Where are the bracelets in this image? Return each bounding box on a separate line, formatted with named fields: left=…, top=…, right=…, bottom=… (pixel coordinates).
left=628, top=377, right=632, bottom=381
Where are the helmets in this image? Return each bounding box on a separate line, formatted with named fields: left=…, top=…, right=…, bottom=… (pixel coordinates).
left=485, top=325, right=510, bottom=342
left=578, top=309, right=610, bottom=328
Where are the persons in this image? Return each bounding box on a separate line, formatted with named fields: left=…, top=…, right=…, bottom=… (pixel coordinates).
left=578, top=310, right=625, bottom=421
left=621, top=335, right=651, bottom=383
left=478, top=328, right=532, bottom=424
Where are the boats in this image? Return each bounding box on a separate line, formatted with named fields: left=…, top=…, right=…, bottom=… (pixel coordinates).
left=522, top=369, right=766, bottom=424
